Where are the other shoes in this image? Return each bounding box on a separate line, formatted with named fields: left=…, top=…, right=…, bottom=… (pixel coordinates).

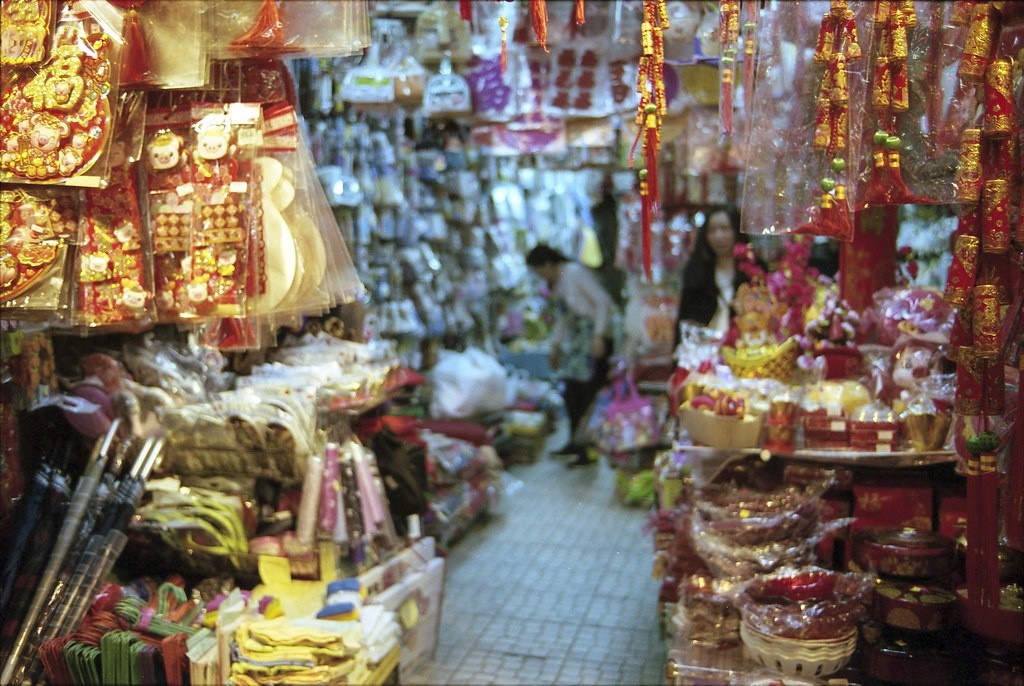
left=550, top=442, right=597, bottom=469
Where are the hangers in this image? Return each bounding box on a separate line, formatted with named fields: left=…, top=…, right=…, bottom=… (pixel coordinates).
left=37, top=571, right=206, bottom=686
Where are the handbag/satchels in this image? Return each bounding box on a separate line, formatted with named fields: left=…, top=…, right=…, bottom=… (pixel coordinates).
left=340, top=38, right=471, bottom=121
left=581, top=373, right=661, bottom=471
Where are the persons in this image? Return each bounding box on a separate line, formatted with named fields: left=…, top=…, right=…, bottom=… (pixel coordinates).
left=525, top=242, right=622, bottom=468
left=671, top=202, right=771, bottom=373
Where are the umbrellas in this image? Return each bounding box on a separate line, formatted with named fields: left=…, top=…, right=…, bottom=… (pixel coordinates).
left=1, top=418, right=174, bottom=685
